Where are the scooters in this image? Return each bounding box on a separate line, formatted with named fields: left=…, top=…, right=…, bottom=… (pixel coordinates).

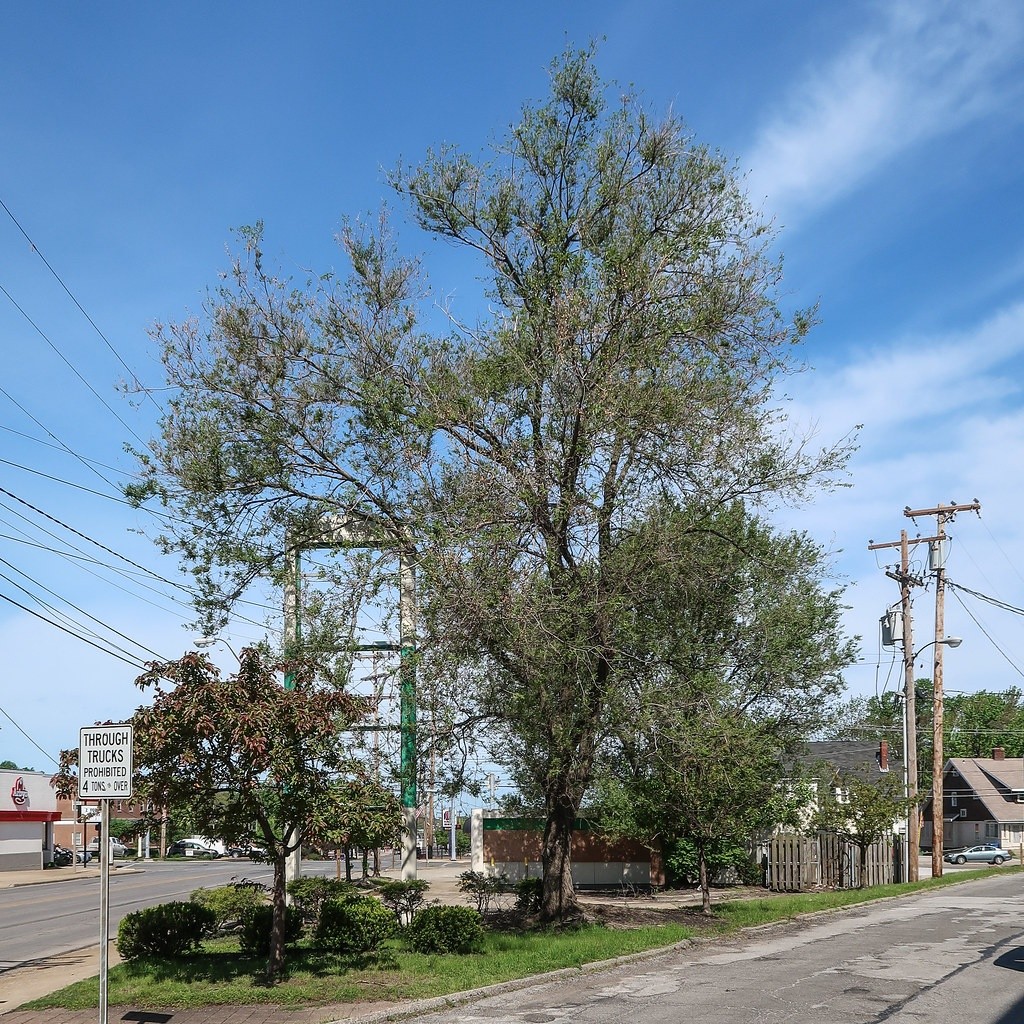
left=46, top=843, right=74, bottom=869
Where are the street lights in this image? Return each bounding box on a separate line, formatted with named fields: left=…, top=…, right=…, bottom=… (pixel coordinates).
left=906, top=636, right=966, bottom=885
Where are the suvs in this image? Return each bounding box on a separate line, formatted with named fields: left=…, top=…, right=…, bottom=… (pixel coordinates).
left=168, top=840, right=219, bottom=859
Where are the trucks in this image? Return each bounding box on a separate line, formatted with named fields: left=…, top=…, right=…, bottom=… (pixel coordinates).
left=73, top=836, right=130, bottom=857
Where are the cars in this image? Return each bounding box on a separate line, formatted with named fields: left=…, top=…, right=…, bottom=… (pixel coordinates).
left=229, top=846, right=263, bottom=859
left=942, top=844, right=1013, bottom=865
left=72, top=850, right=92, bottom=864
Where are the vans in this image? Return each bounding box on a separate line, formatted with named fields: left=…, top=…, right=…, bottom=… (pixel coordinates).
left=177, top=838, right=228, bottom=857
